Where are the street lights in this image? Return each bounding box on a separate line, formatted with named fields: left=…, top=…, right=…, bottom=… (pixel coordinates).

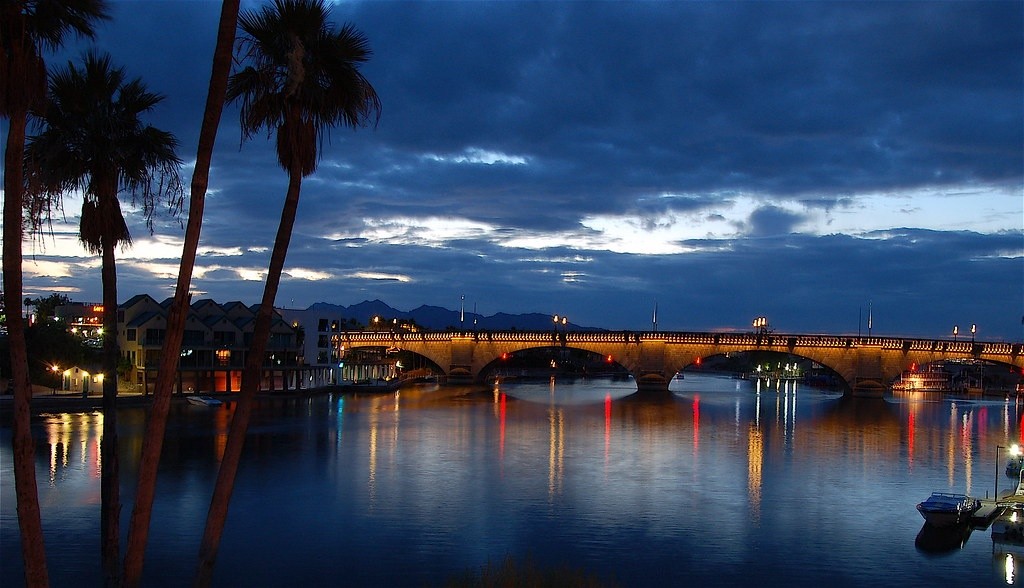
left=994, top=445, right=1018, bottom=504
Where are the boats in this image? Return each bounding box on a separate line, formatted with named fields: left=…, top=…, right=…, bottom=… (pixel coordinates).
left=674, top=372, right=685, bottom=379
left=186, top=395, right=222, bottom=408
left=915, top=490, right=982, bottom=525
